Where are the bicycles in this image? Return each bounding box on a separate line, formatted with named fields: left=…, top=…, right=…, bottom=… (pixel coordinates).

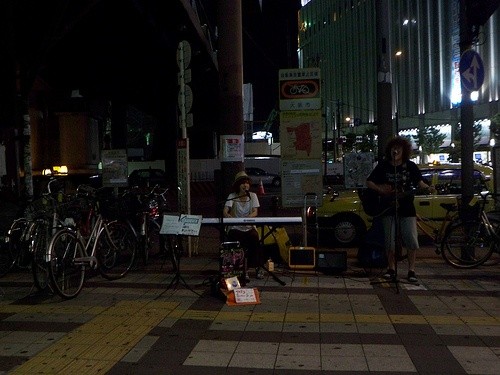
left=0, top=169, right=185, bottom=300
left=411, top=190, right=500, bottom=270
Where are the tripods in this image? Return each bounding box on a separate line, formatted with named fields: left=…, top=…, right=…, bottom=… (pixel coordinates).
left=153, top=214, right=205, bottom=300
left=370, top=154, right=420, bottom=295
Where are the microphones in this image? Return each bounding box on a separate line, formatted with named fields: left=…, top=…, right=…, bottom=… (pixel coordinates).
left=245, top=190, right=251, bottom=200
left=394, top=150, right=397, bottom=154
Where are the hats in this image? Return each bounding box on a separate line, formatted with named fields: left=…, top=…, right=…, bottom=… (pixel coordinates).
left=235, top=171, right=251, bottom=183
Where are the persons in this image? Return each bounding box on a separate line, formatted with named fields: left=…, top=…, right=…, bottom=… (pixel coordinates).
left=367, top=138, right=435, bottom=283
left=223, top=171, right=260, bottom=282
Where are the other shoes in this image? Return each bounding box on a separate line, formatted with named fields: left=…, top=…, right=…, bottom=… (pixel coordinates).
left=256, top=268, right=265, bottom=279
left=407, top=271, right=417, bottom=281
left=383, top=269, right=395, bottom=277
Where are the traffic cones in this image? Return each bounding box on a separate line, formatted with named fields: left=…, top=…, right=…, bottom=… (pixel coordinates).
left=255, top=177, right=268, bottom=196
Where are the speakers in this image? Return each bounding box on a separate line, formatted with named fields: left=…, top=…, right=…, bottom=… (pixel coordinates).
left=288, top=247, right=347, bottom=276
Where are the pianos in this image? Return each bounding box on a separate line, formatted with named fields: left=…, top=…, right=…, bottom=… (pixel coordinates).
left=201, top=217, right=303, bottom=287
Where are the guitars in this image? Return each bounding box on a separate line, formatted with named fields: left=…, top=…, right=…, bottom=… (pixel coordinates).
left=361, top=182, right=451, bottom=218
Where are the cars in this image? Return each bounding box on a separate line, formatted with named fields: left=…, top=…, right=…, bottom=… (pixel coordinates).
left=306, top=161, right=496, bottom=247
left=245, top=167, right=281, bottom=187
